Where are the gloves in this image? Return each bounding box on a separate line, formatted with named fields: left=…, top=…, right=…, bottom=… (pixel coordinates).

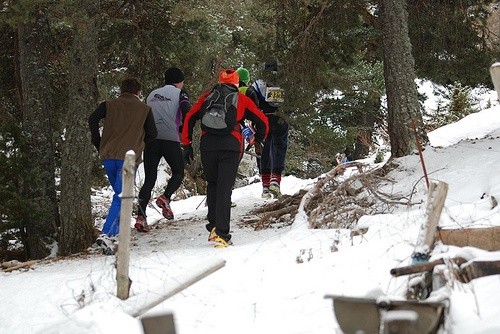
left=183, top=146, right=194, bottom=165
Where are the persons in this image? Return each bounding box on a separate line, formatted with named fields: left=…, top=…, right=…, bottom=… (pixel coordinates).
left=88, top=78, right=157, bottom=255
left=182, top=67, right=269, bottom=248
left=250, top=58, right=288, bottom=199
left=134, top=67, right=196, bottom=232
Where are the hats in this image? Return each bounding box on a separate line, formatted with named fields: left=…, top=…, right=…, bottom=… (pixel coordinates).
left=236, top=68, right=250, bottom=84
left=164, top=67, right=184, bottom=84
left=219, top=69, right=239, bottom=88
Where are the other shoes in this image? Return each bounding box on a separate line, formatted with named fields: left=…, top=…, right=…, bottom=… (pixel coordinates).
left=231, top=202, right=237, bottom=207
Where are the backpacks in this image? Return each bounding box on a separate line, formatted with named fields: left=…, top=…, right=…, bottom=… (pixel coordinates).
left=256, top=82, right=288, bottom=140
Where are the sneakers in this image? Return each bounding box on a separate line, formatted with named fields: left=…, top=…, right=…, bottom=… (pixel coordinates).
left=135, top=216, right=150, bottom=233
left=269, top=184, right=282, bottom=200
left=208, top=227, right=217, bottom=241
left=262, top=192, right=271, bottom=200
left=96, top=233, right=118, bottom=256
left=214, top=239, right=233, bottom=248
left=156, top=196, right=174, bottom=220
